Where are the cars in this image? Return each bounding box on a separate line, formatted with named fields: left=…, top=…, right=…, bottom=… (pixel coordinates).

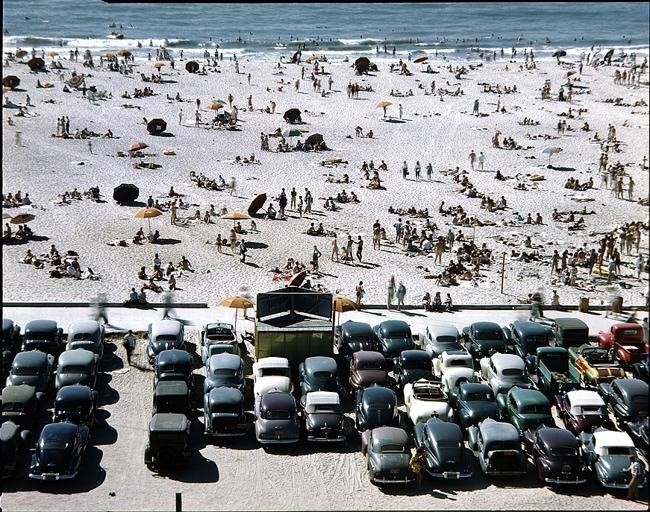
left=431, top=352, right=476, bottom=390
left=6, top=348, right=54, bottom=390
left=0, top=319, right=20, bottom=369
left=300, top=391, right=346, bottom=441
left=55, top=348, right=99, bottom=389
left=462, top=321, right=507, bottom=357
left=336, top=321, right=373, bottom=355
left=152, top=380, right=192, bottom=417
left=412, top=416, right=473, bottom=479
left=204, top=352, right=246, bottom=393
left=522, top=424, right=587, bottom=483
left=547, top=318, right=592, bottom=347
left=468, top=417, right=527, bottom=475
left=299, top=356, right=337, bottom=394
left=54, top=382, right=98, bottom=423
left=22, top=320, right=63, bottom=353
left=146, top=320, right=184, bottom=359
left=28, top=423, right=91, bottom=481
left=502, top=319, right=551, bottom=357
left=579, top=426, right=643, bottom=488
left=0, top=421, right=32, bottom=479
left=144, top=412, right=190, bottom=464
left=479, top=352, right=536, bottom=394
left=204, top=386, right=246, bottom=436
left=0, top=384, right=45, bottom=423
left=371, top=320, right=413, bottom=358
left=255, top=391, right=300, bottom=443
left=450, top=384, right=499, bottom=424
left=498, top=386, right=556, bottom=431
left=348, top=351, right=389, bottom=389
left=361, top=425, right=416, bottom=483
left=153, top=347, right=194, bottom=389
left=598, top=379, right=650, bottom=442
left=394, top=349, right=433, bottom=386
left=66, top=320, right=105, bottom=359
left=553, top=391, right=615, bottom=436
left=419, top=323, right=462, bottom=356
left=356, top=383, right=400, bottom=429
left=252, top=356, right=294, bottom=398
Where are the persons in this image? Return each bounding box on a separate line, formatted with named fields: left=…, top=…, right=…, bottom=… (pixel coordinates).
left=140, top=73, right=161, bottom=83
left=643, top=317, right=650, bottom=342
left=419, top=81, right=463, bottom=101
left=2, top=187, right=31, bottom=207
left=55, top=115, right=113, bottom=154
left=122, top=87, right=153, bottom=98
left=555, top=108, right=589, bottom=134
left=60, top=186, right=100, bottom=204
left=323, top=157, right=433, bottom=190
left=14, top=130, right=25, bottom=148
left=391, top=89, right=413, bottom=98
left=325, top=191, right=358, bottom=211
left=622, top=455, right=641, bottom=500
left=274, top=52, right=334, bottom=97
left=330, top=219, right=384, bottom=265
left=190, top=171, right=238, bottom=196
left=163, top=185, right=188, bottom=224
left=519, top=118, right=540, bottom=126
left=4, top=47, right=104, bottom=73
left=513, top=235, right=596, bottom=290
left=59, top=71, right=111, bottom=103
left=148, top=45, right=183, bottom=62
left=107, top=55, right=135, bottom=75
left=232, top=53, right=252, bottom=84
left=260, top=128, right=325, bottom=153
left=151, top=231, right=159, bottom=240
left=597, top=222, right=650, bottom=288
left=409, top=448, right=424, bottom=490
left=390, top=59, right=412, bottom=77
left=491, top=129, right=520, bottom=151
left=122, top=329, right=137, bottom=365
left=515, top=183, right=526, bottom=192
left=236, top=152, right=256, bottom=164
left=398, top=104, right=403, bottom=119
left=137, top=228, right=142, bottom=238
left=593, top=125, right=650, bottom=206
left=211, top=111, right=241, bottom=132
left=542, top=80, right=573, bottom=102
left=480, top=48, right=537, bottom=71
left=3, top=224, right=36, bottom=239
left=2, top=95, right=33, bottom=126
left=440, top=151, right=507, bottom=228
left=386, top=206, right=433, bottom=251
left=526, top=207, right=587, bottom=232
left=167, top=91, right=201, bottom=127
left=603, top=97, right=647, bottom=107
left=216, top=221, right=257, bottom=263
left=345, top=55, right=378, bottom=76
left=130, top=253, right=192, bottom=304
left=347, top=82, right=372, bottom=99
left=148, top=195, right=163, bottom=209
left=477, top=82, right=517, bottom=94
left=25, top=245, right=99, bottom=280
left=355, top=276, right=452, bottom=313
left=582, top=440, right=594, bottom=471
left=270, top=246, right=330, bottom=294
left=472, top=100, right=506, bottom=117
left=267, top=188, right=313, bottom=215
left=566, top=177, right=593, bottom=191
left=432, top=230, right=493, bottom=286
left=140, top=117, right=147, bottom=126
left=347, top=124, right=375, bottom=142
left=200, top=49, right=223, bottom=72
left=577, top=45, right=647, bottom=86
left=427, top=63, right=484, bottom=80
left=228, top=93, right=277, bottom=114
left=190, top=204, right=218, bottom=224
left=118, top=142, right=156, bottom=170
left=35, top=80, right=44, bottom=88
left=520, top=291, right=561, bottom=322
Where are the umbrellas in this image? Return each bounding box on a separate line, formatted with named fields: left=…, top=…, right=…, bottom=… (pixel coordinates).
left=222, top=212, right=249, bottom=225
left=552, top=48, right=566, bottom=57
left=186, top=61, right=199, bottom=73
left=207, top=103, right=224, bottom=109
left=113, top=183, right=138, bottom=201
left=332, top=297, right=359, bottom=325
left=154, top=62, right=165, bottom=68
left=562, top=70, right=577, bottom=80
left=10, top=214, right=37, bottom=227
left=118, top=48, right=129, bottom=55
left=248, top=194, right=267, bottom=216
left=146, top=118, right=167, bottom=132
left=211, top=99, right=226, bottom=104
left=2, top=75, right=20, bottom=88
left=376, top=100, right=393, bottom=109
left=414, top=56, right=428, bottom=63
left=283, top=108, right=301, bottom=123
left=135, top=206, right=162, bottom=237
left=543, top=146, right=563, bottom=153
left=218, top=295, right=253, bottom=325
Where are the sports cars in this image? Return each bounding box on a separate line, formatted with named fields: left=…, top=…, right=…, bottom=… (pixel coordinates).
left=568, top=344, right=625, bottom=385
left=404, top=378, right=454, bottom=424
left=201, top=322, right=241, bottom=364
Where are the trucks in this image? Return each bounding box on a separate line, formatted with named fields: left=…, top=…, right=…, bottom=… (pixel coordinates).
left=535, top=346, right=581, bottom=389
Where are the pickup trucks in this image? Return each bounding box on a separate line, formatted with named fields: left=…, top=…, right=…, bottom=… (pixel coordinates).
left=598, top=323, right=650, bottom=365
